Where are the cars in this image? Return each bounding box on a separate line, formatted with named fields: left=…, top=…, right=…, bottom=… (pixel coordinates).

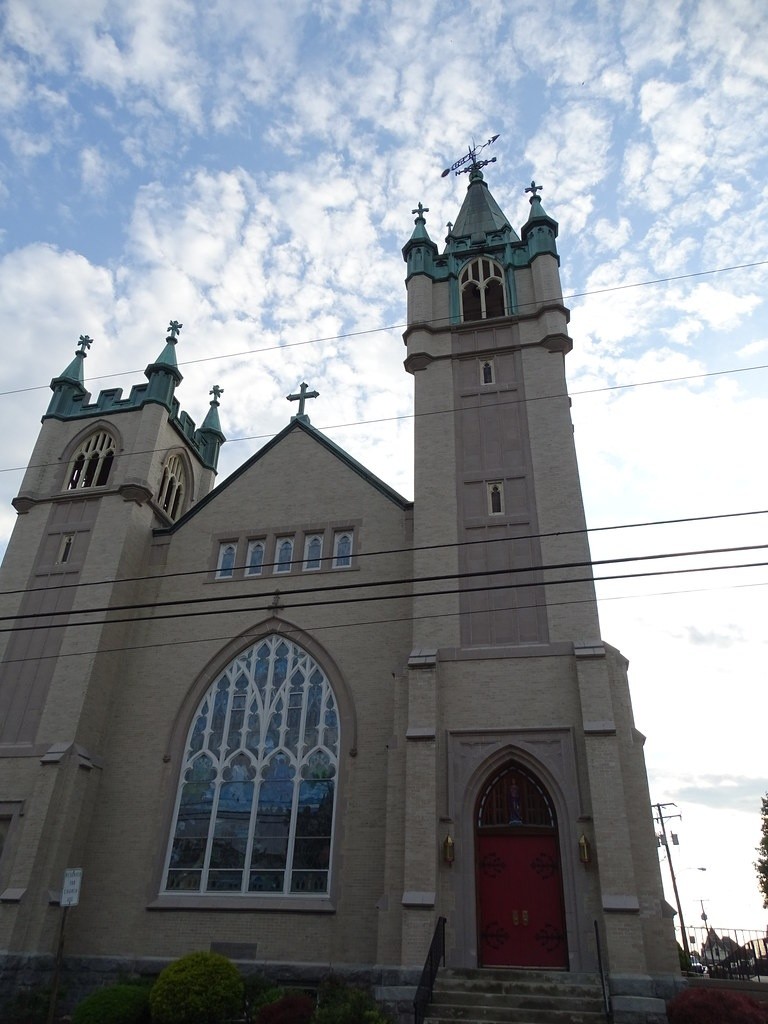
left=690, top=955, right=708, bottom=973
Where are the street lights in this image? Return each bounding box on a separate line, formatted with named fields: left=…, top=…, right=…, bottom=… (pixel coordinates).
left=672, top=867, right=707, bottom=960
